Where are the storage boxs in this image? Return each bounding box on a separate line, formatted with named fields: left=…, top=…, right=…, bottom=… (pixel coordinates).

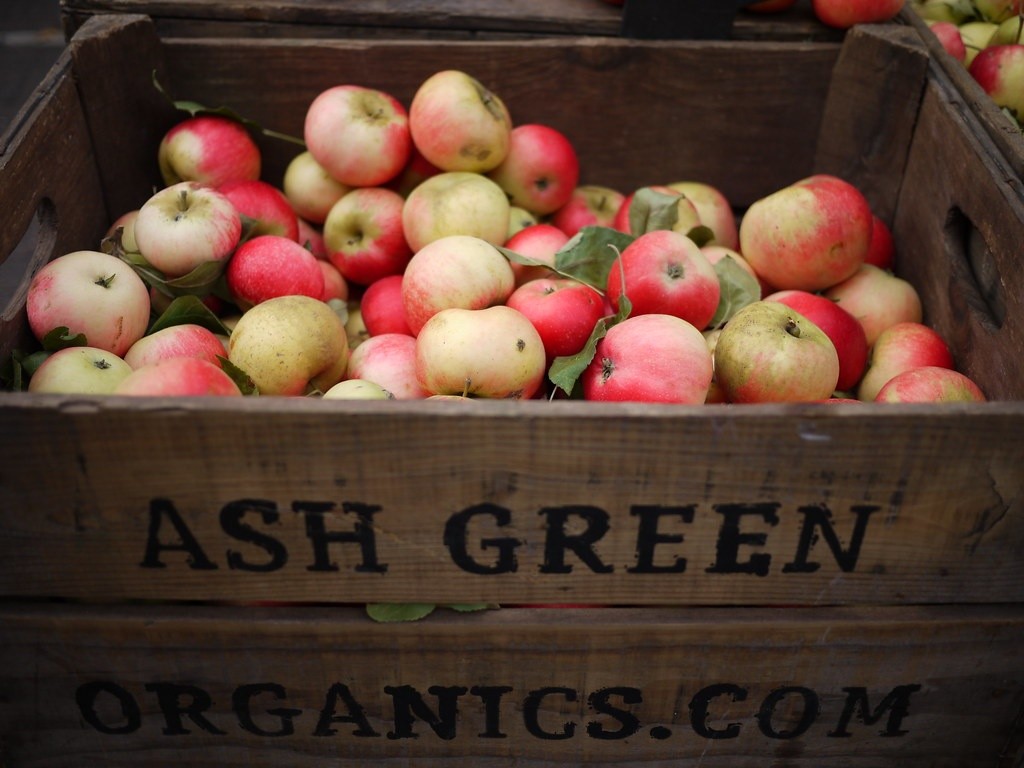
left=904, top=0, right=1024, bottom=184
left=0, top=600, right=1024, bottom=768
left=47, top=0, right=859, bottom=45
left=0, top=11, right=1024, bottom=608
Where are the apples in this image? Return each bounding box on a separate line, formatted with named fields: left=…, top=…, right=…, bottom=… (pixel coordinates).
left=25, top=70, right=987, bottom=405
left=743, top=0, right=1024, bottom=133
